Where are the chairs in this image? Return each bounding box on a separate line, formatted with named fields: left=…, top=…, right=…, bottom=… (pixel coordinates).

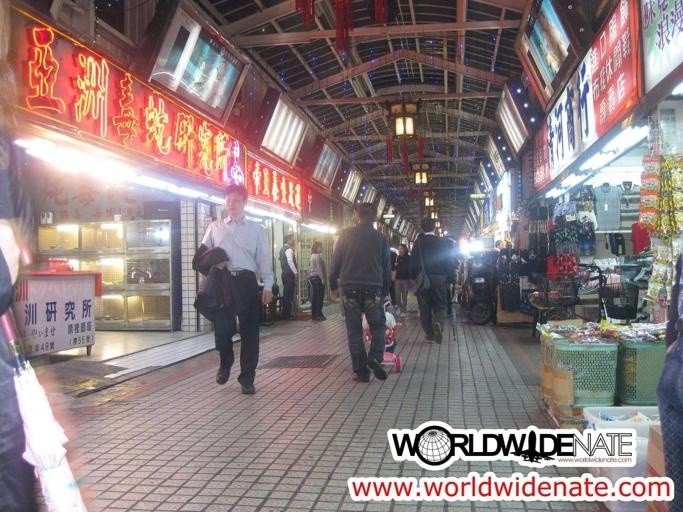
left=530, top=272, right=579, bottom=341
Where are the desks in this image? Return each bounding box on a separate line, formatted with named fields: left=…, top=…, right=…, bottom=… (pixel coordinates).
left=12, top=270, right=102, bottom=358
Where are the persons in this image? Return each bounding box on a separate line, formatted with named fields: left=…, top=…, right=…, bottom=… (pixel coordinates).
left=0, top=57, right=44, bottom=512
left=658, top=248, right=683, bottom=511
left=196, top=185, right=504, bottom=397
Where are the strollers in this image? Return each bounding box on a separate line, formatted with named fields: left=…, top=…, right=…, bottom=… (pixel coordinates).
left=362, top=298, right=402, bottom=374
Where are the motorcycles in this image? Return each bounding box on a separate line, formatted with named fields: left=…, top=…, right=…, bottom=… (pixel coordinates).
left=457, top=256, right=493, bottom=323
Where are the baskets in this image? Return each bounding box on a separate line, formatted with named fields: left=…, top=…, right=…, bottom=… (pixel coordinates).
left=536, top=271, right=581, bottom=307
left=547, top=336, right=668, bottom=409
left=600, top=283, right=639, bottom=319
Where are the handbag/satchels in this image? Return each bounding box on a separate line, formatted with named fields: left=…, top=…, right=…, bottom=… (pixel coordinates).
left=409, top=272, right=431, bottom=296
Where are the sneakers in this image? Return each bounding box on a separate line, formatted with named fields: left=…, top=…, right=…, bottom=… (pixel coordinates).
left=216, top=366, right=230, bottom=384
left=237, top=376, right=256, bottom=394
left=431, top=322, right=443, bottom=345
left=367, top=356, right=388, bottom=380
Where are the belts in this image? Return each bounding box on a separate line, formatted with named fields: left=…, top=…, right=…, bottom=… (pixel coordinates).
left=229, top=269, right=249, bottom=277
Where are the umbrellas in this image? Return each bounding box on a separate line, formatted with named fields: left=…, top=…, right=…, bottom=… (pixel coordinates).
left=0, top=235, right=91, bottom=511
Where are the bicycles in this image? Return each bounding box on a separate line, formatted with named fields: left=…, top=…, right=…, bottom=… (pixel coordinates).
left=529, top=259, right=640, bottom=339
